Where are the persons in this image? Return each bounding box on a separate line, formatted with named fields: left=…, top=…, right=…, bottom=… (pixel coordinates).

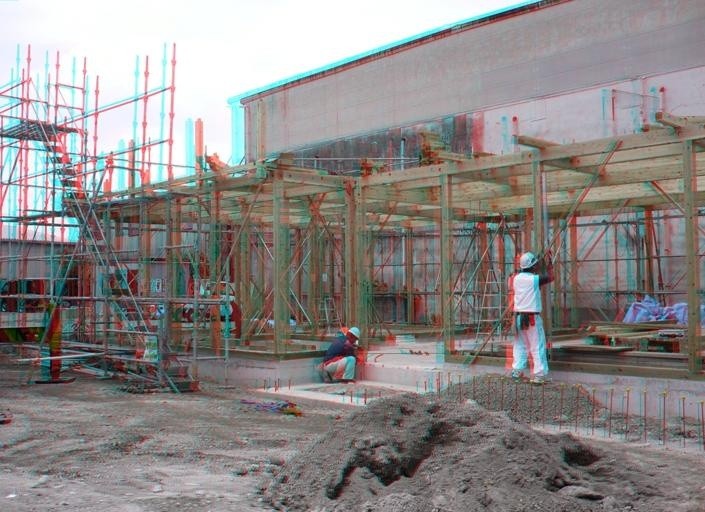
left=319, top=327, right=361, bottom=384
left=512, top=252, right=555, bottom=385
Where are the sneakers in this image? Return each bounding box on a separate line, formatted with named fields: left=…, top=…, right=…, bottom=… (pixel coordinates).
left=513, top=370, right=553, bottom=384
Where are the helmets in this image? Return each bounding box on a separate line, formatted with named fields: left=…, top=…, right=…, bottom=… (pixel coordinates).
left=347, top=327, right=362, bottom=340
left=520, top=252, right=539, bottom=269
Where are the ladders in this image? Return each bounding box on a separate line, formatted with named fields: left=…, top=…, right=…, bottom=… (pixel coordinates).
left=476, top=270, right=503, bottom=343
